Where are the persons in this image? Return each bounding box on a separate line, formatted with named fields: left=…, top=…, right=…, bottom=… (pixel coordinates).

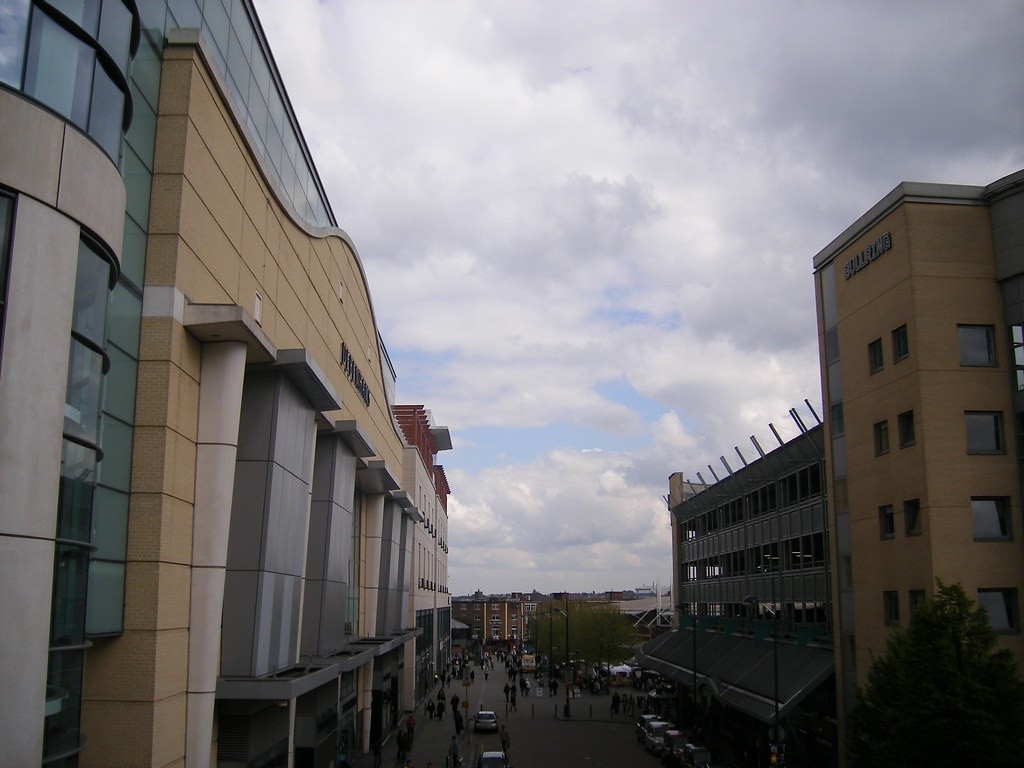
left=450, top=694, right=459, bottom=712
left=500, top=724, right=510, bottom=750
left=470, top=670, right=474, bottom=684
left=509, top=682, right=518, bottom=696
left=503, top=682, right=510, bottom=702
left=510, top=693, right=517, bottom=712
left=370, top=648, right=747, bottom=768
left=452, top=735, right=458, bottom=767
left=454, top=710, right=463, bottom=735
left=451, top=667, right=456, bottom=679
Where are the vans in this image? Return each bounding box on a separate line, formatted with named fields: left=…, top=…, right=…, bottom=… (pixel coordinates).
left=521, top=654, right=537, bottom=674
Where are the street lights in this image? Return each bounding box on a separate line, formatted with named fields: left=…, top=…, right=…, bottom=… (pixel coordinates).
left=539, top=600, right=558, bottom=720
left=531, top=606, right=539, bottom=678
left=554, top=595, right=570, bottom=719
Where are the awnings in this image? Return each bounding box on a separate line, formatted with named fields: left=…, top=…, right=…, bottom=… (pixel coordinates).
left=638, top=627, right=835, bottom=725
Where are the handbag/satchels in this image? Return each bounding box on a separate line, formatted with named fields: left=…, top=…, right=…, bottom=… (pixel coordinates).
left=460, top=724, right=464, bottom=729
left=507, top=742, right=510, bottom=749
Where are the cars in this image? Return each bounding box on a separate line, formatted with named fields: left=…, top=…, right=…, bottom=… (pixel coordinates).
left=478, top=750, right=513, bottom=768
left=635, top=713, right=724, bottom=768
left=590, top=659, right=614, bottom=677
left=473, top=710, right=499, bottom=734
